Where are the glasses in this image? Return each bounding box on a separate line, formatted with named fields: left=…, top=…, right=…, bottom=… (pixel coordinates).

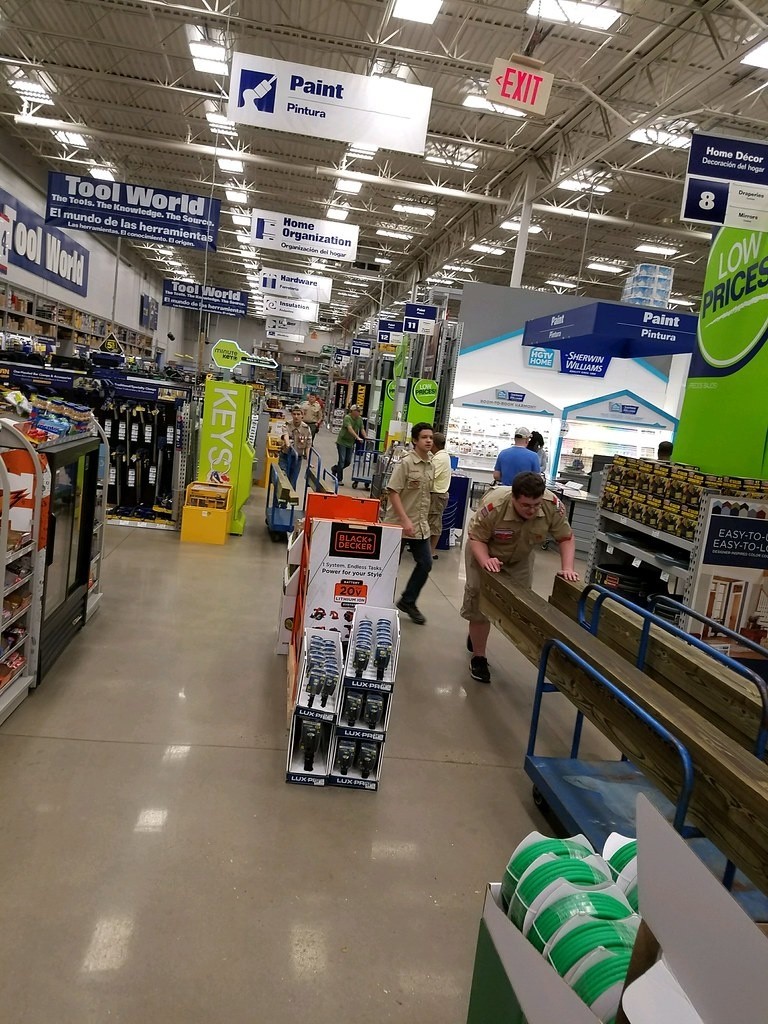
left=515, top=500, right=542, bottom=510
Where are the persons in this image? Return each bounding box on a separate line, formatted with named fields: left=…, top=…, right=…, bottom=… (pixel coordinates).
left=493, top=427, right=540, bottom=486
left=526, top=431, right=548, bottom=471
left=281, top=404, right=312, bottom=493
left=331, top=404, right=373, bottom=486
left=406, top=432, right=451, bottom=560
left=300, top=391, right=325, bottom=448
left=657, top=441, right=674, bottom=461
left=386, top=422, right=434, bottom=624
left=460, top=472, right=581, bottom=683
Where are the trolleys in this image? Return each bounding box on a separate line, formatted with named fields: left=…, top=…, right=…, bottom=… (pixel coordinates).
left=351, top=437, right=385, bottom=489
left=265, top=445, right=338, bottom=533
left=524, top=584, right=768, bottom=923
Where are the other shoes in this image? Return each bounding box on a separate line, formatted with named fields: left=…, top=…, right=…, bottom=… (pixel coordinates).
left=338, top=482, right=344, bottom=486
left=331, top=465, right=337, bottom=480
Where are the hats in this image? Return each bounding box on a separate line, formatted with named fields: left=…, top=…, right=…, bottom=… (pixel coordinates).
left=350, top=404, right=363, bottom=411
left=515, top=427, right=533, bottom=438
left=291, top=404, right=304, bottom=416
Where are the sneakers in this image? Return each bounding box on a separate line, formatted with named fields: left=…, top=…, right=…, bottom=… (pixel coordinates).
left=396, top=597, right=424, bottom=624
left=467, top=635, right=473, bottom=652
left=469, top=656, right=491, bottom=682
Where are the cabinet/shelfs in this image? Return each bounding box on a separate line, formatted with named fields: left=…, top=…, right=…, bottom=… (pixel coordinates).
left=591, top=462, right=768, bottom=661
left=0, top=419, right=43, bottom=727
left=0, top=277, right=155, bottom=359
left=250, top=346, right=323, bottom=396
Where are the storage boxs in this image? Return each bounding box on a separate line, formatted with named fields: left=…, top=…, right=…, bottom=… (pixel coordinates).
left=464, top=790, right=767, bottom=1024
left=284, top=516, right=405, bottom=791
